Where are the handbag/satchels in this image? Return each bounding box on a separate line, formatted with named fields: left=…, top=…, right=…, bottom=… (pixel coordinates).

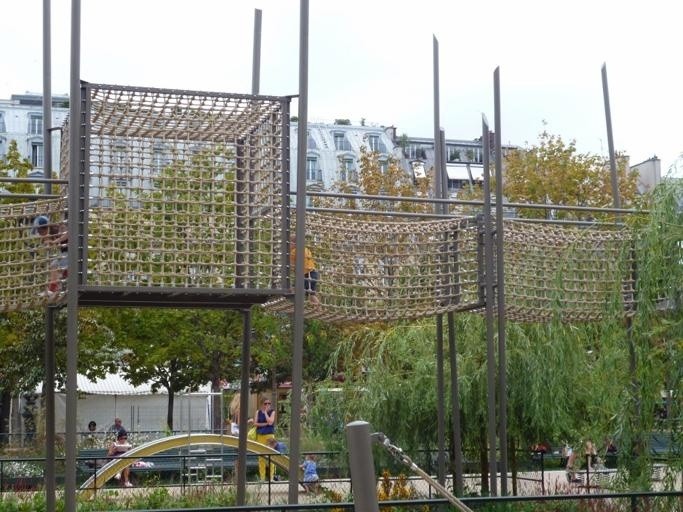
left=248, top=424, right=258, bottom=442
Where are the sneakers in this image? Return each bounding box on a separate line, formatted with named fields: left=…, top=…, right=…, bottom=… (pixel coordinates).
left=114, top=472, right=122, bottom=480
left=124, top=481, right=133, bottom=487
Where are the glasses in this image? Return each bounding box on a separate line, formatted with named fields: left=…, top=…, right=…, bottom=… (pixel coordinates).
left=119, top=437, right=128, bottom=440
left=263, top=402, right=272, bottom=407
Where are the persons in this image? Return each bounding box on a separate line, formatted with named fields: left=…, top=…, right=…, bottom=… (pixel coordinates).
left=660, top=406, right=667, bottom=419
left=299, top=455, right=319, bottom=495
left=255, top=397, right=276, bottom=482
left=224, top=418, right=256, bottom=440
left=82, top=420, right=97, bottom=438
left=213, top=374, right=261, bottom=392
left=274, top=442, right=288, bottom=480
left=30, top=215, right=69, bottom=295
left=289, top=235, right=320, bottom=303
left=108, top=432, right=134, bottom=488
left=532, top=437, right=642, bottom=484
left=107, top=417, right=127, bottom=443
left=654, top=404, right=660, bottom=419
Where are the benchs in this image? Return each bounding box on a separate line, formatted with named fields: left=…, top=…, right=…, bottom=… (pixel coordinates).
left=77, top=445, right=276, bottom=490
left=529, top=432, right=682, bottom=467
